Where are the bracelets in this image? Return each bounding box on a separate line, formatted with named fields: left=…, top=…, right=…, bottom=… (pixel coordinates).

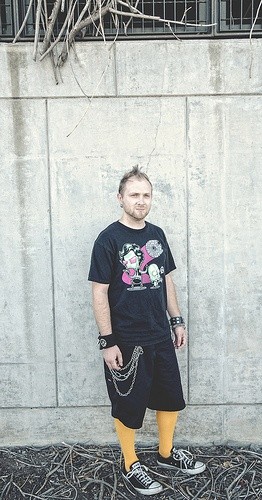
left=170, top=317, right=184, bottom=325
left=98, top=333, right=117, bottom=350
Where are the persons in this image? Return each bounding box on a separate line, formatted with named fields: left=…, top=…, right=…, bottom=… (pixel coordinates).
left=88, top=164, right=206, bottom=495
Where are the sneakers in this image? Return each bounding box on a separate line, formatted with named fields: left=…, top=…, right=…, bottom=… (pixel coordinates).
left=157, top=446, right=206, bottom=475
left=121, top=461, right=163, bottom=495
